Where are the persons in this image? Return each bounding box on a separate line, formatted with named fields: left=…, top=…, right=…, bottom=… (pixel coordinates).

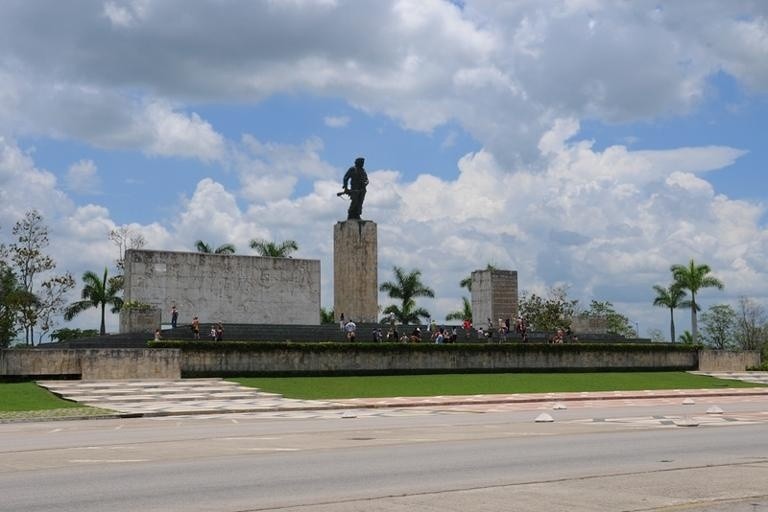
left=208, top=325, right=217, bottom=341
left=191, top=317, right=200, bottom=341
left=339, top=312, right=577, bottom=344
left=215, top=321, right=224, bottom=341
left=154, top=329, right=163, bottom=342
left=343, top=158, right=369, bottom=220
left=170, top=307, right=179, bottom=328
